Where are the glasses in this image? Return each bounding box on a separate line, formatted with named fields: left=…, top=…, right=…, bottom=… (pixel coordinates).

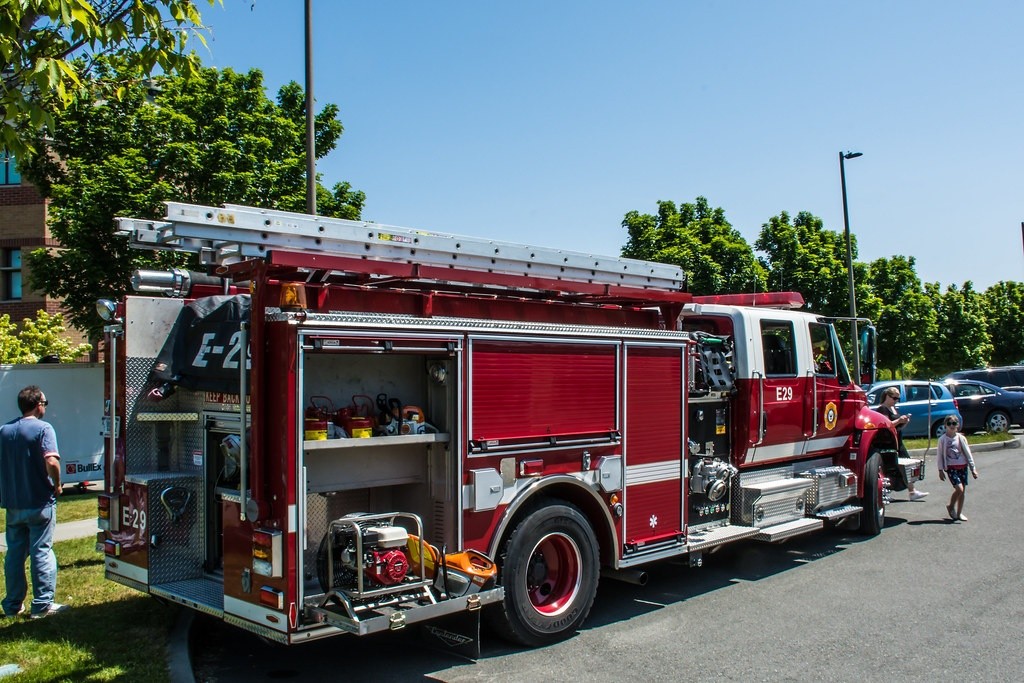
left=38, top=400, right=49, bottom=407
left=888, top=394, right=901, bottom=401
left=947, top=421, right=959, bottom=426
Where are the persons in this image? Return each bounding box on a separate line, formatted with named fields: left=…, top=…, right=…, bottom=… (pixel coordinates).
left=937, top=415, right=978, bottom=521
left=0, top=387, right=70, bottom=616
left=876, top=386, right=930, bottom=501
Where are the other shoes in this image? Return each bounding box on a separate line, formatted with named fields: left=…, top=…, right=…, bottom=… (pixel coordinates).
left=31, top=602, right=70, bottom=619
left=946, top=504, right=958, bottom=520
left=5, top=602, right=25, bottom=617
left=956, top=513, right=968, bottom=521
left=910, top=489, right=929, bottom=501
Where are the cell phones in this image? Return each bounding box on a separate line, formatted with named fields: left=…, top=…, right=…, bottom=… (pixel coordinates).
left=907, top=413, right=912, bottom=417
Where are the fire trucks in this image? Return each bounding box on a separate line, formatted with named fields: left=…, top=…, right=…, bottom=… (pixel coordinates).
left=83, top=197, right=928, bottom=663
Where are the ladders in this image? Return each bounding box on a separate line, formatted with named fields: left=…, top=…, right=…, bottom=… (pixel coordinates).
left=112, top=200, right=685, bottom=290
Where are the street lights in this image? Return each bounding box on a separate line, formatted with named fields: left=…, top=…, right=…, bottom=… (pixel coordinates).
left=838, top=147, right=867, bottom=385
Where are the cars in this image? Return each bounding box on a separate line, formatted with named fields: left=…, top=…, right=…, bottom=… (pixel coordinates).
left=920, top=378, right=1024, bottom=435
left=860, top=380, right=964, bottom=441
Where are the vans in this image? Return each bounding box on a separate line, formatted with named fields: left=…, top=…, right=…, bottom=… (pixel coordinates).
left=941, top=366, right=1024, bottom=392
